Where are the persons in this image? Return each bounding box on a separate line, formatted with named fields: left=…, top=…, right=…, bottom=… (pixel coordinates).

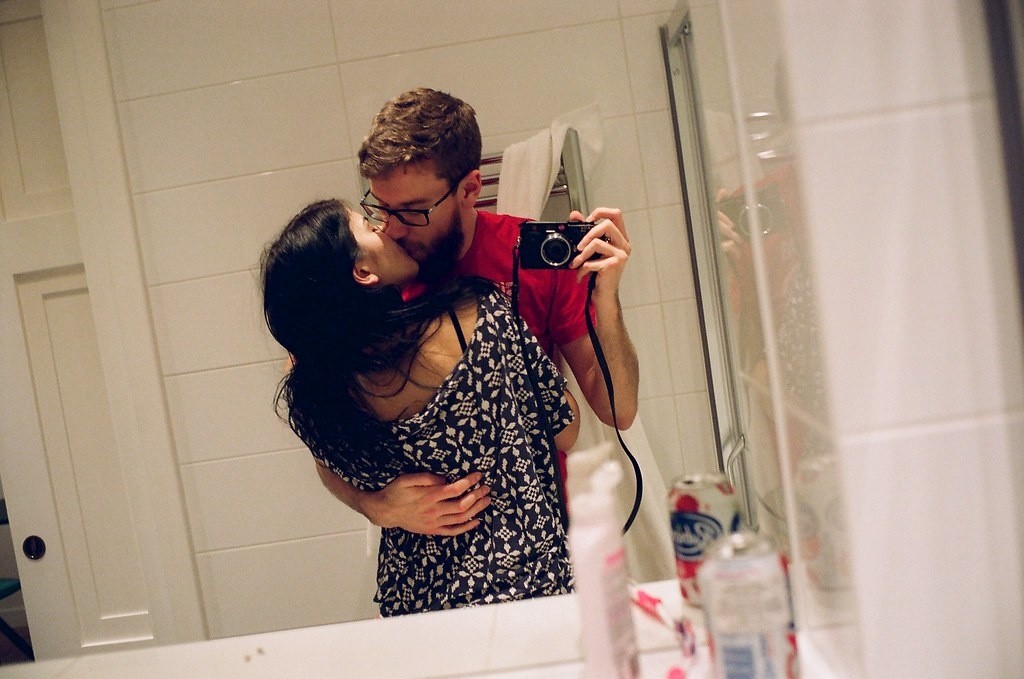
left=282, top=85, right=641, bottom=536
left=716, top=57, right=856, bottom=611
left=258, top=200, right=582, bottom=615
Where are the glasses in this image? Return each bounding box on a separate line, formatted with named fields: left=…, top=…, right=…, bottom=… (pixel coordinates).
left=360, top=181, right=459, bottom=227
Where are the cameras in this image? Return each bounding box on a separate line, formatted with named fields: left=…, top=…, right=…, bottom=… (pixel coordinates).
left=518, top=221, right=607, bottom=270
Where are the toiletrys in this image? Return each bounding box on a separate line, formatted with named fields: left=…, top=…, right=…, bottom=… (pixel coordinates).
left=565, top=458, right=640, bottom=679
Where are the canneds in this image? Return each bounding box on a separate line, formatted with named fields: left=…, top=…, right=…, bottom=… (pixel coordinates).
left=698, top=530, right=802, bottom=679
left=666, top=472, right=746, bottom=608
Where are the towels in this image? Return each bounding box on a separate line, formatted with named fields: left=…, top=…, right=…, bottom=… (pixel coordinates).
left=496, top=101, right=605, bottom=223
